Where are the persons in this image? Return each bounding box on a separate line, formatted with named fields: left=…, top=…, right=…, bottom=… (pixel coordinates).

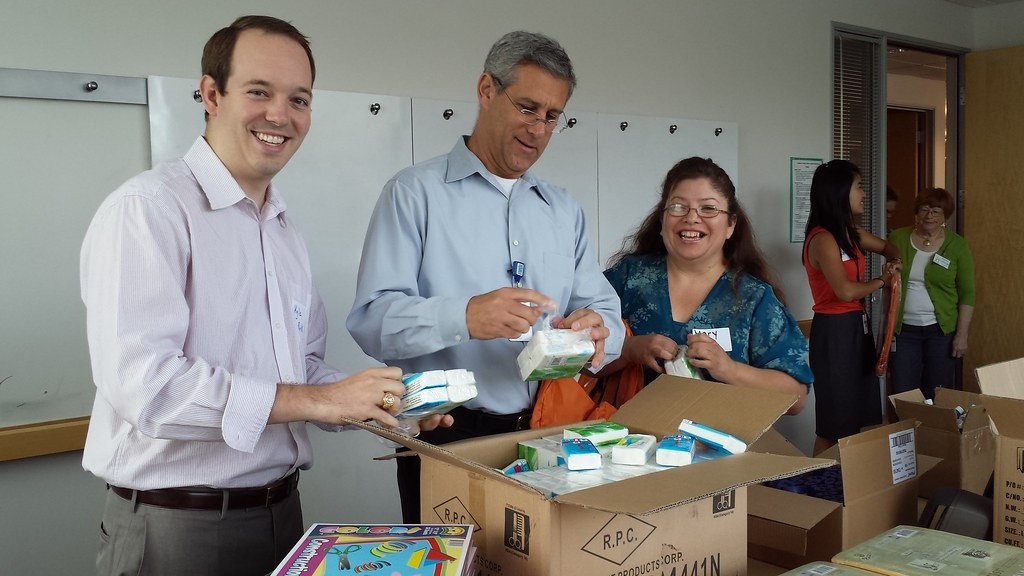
left=603, top=156, right=814, bottom=416
left=886, top=184, right=897, bottom=238
left=886, top=187, right=975, bottom=403
left=81, top=15, right=454, bottom=576
left=802, top=159, right=903, bottom=457
left=346, top=30, right=626, bottom=526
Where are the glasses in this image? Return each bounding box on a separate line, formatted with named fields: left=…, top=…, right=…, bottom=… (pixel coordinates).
left=660, top=203, right=732, bottom=217
left=917, top=207, right=944, bottom=216
left=492, top=75, right=560, bottom=126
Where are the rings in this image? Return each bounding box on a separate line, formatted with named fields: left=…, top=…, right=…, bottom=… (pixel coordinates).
left=413, top=432, right=419, bottom=437
left=380, top=392, right=395, bottom=410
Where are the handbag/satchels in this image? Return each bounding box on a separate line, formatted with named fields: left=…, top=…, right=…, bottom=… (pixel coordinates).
left=528, top=319, right=644, bottom=432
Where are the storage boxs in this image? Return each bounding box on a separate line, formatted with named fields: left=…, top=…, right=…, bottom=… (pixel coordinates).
left=973, top=357, right=1024, bottom=549
left=337, top=369, right=837, bottom=576
left=887, top=384, right=1000, bottom=499
left=737, top=418, right=946, bottom=569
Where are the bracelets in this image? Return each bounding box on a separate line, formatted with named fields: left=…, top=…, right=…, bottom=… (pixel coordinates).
left=873, top=278, right=885, bottom=289
left=886, top=256, right=901, bottom=263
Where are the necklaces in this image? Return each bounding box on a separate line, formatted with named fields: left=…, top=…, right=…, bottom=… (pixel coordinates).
left=916, top=228, right=942, bottom=247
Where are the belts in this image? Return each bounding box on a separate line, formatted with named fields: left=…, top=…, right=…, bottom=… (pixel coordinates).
left=109, top=469, right=298, bottom=509
left=446, top=408, right=532, bottom=434
